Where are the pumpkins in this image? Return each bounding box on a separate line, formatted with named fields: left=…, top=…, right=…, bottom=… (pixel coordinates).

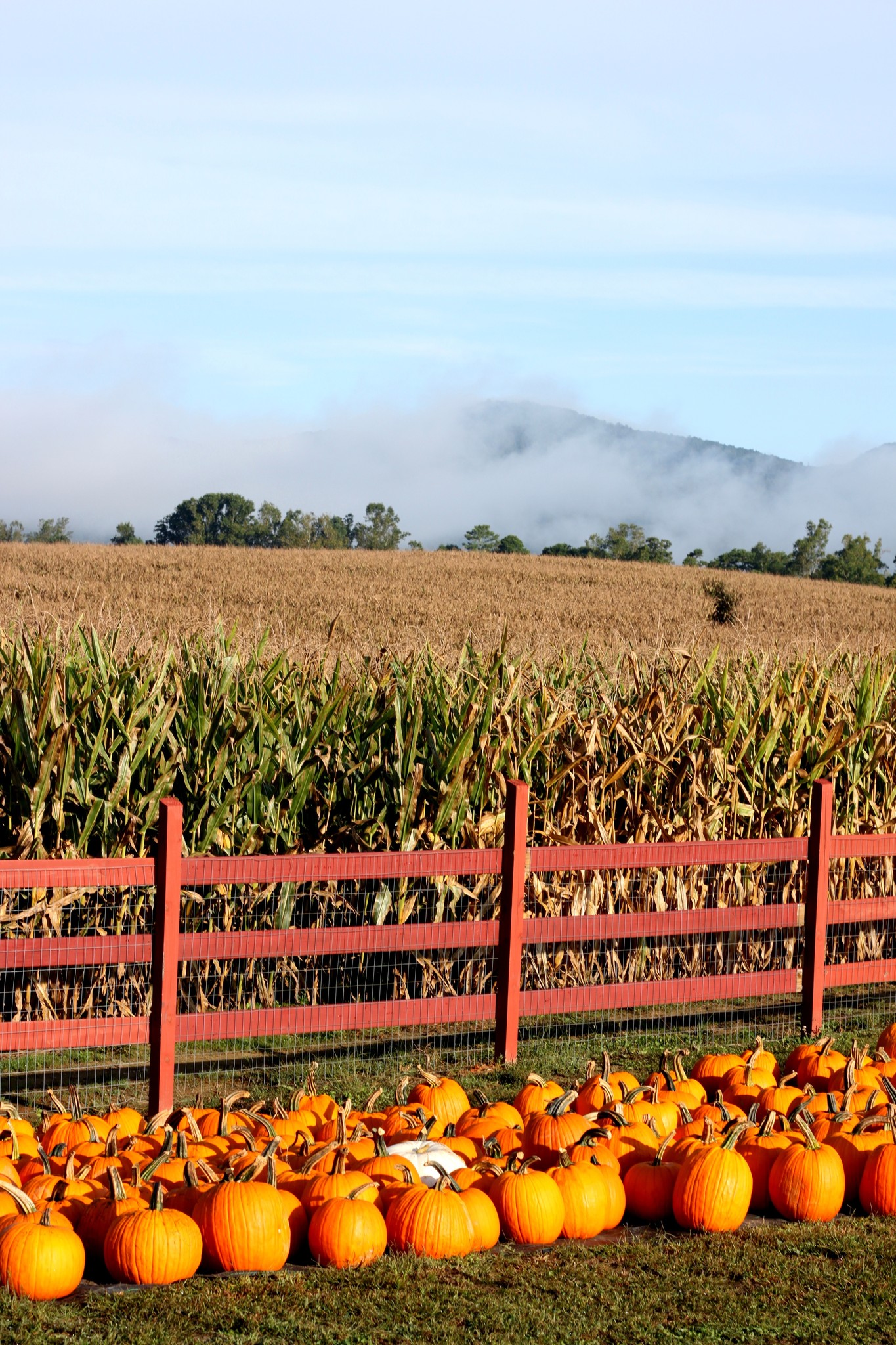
left=453, top=1019, right=896, bottom=1247
left=0, top=1061, right=502, bottom=1300
